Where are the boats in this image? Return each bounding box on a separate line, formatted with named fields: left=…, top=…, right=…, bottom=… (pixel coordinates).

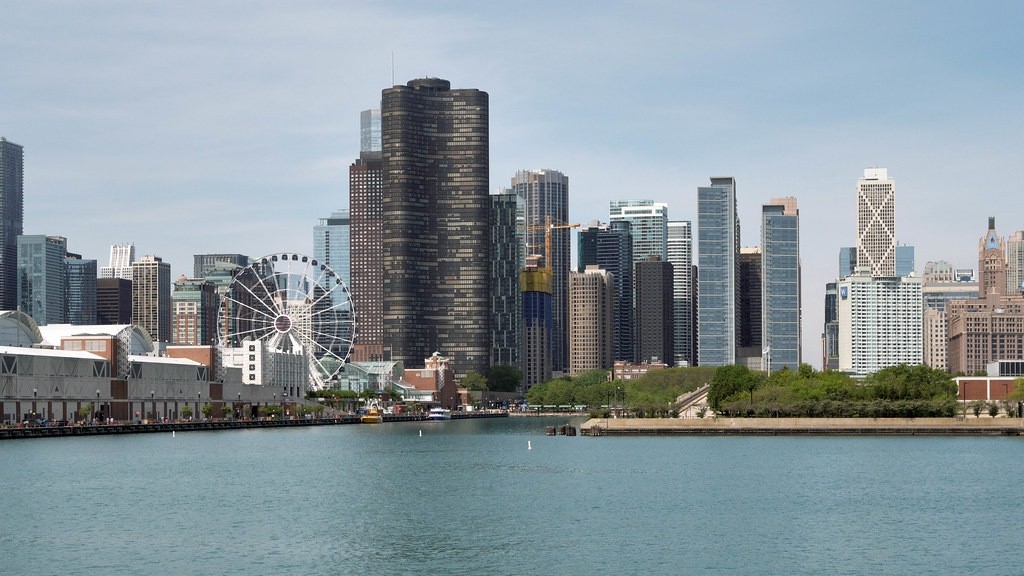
left=429, top=407, right=451, bottom=419
left=361, top=409, right=383, bottom=423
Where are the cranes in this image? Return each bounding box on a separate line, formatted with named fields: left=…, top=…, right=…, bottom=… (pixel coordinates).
left=516, top=215, right=581, bottom=272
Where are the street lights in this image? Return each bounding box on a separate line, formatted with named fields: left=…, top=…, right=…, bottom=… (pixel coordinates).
left=304, top=393, right=308, bottom=418
left=96, top=390, right=101, bottom=426
left=237, top=392, right=242, bottom=420
left=1002, top=384, right=1008, bottom=405
left=151, top=390, right=155, bottom=424
left=33, top=389, right=38, bottom=428
left=198, top=392, right=202, bottom=421
left=273, top=392, right=276, bottom=420
left=963, top=381, right=968, bottom=417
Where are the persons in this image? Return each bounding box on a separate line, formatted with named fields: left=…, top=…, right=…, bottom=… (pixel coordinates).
left=148, top=413, right=315, bottom=423
left=23, top=417, right=114, bottom=428
